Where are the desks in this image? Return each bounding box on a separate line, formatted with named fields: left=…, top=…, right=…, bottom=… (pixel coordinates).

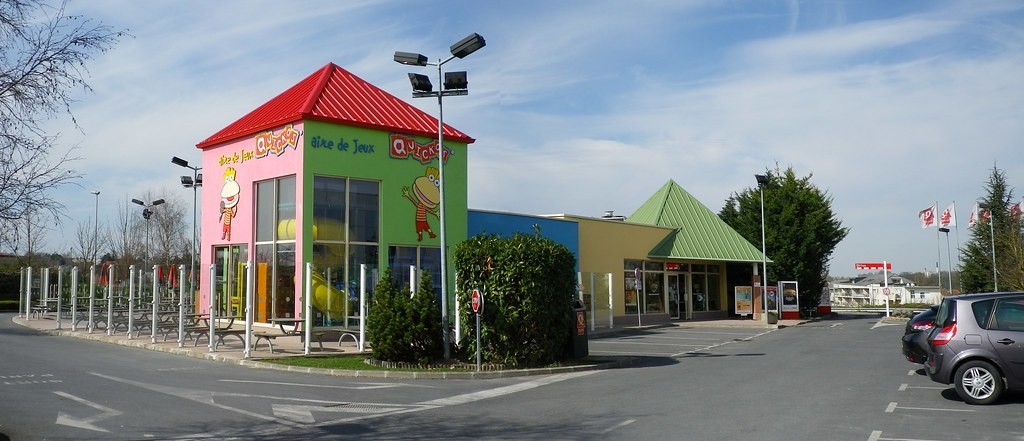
left=348, top=316, right=367, bottom=330
left=78, top=307, right=305, bottom=334
left=39, top=296, right=180, bottom=310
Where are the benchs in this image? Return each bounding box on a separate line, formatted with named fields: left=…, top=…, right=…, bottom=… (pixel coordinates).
left=76, top=316, right=324, bottom=354
left=336, top=330, right=368, bottom=349
left=32, top=305, right=70, bottom=320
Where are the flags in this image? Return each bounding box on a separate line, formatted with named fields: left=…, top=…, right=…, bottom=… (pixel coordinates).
left=981, top=209, right=990, bottom=224
left=918, top=204, right=937, bottom=228
left=969, top=207, right=977, bottom=225
left=939, top=204, right=956, bottom=228
left=1011, top=205, right=1022, bottom=221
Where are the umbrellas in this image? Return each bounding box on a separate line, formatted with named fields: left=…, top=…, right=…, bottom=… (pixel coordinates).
left=158, top=265, right=165, bottom=286
left=98, top=263, right=108, bottom=285
left=188, top=272, right=191, bottom=283
left=168, top=263, right=179, bottom=289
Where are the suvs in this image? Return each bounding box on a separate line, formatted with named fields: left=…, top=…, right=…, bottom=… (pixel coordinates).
left=902, top=304, right=939, bottom=364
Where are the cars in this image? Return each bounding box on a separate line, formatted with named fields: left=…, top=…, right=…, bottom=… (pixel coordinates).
left=923, top=290, right=1024, bottom=405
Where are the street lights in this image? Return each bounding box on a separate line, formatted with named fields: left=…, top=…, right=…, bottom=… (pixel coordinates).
left=939, top=227, right=951, bottom=295
left=172, top=156, right=202, bottom=315
left=393, top=31, right=486, bottom=360
left=91, top=190, right=101, bottom=278
left=755, top=174, right=769, bottom=326
left=132, top=197, right=165, bottom=307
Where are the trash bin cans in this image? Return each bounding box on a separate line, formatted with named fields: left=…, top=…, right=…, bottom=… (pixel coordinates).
left=571, top=299, right=589, bottom=358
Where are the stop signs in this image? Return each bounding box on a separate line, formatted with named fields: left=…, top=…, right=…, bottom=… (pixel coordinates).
left=469, top=289, right=481, bottom=311
